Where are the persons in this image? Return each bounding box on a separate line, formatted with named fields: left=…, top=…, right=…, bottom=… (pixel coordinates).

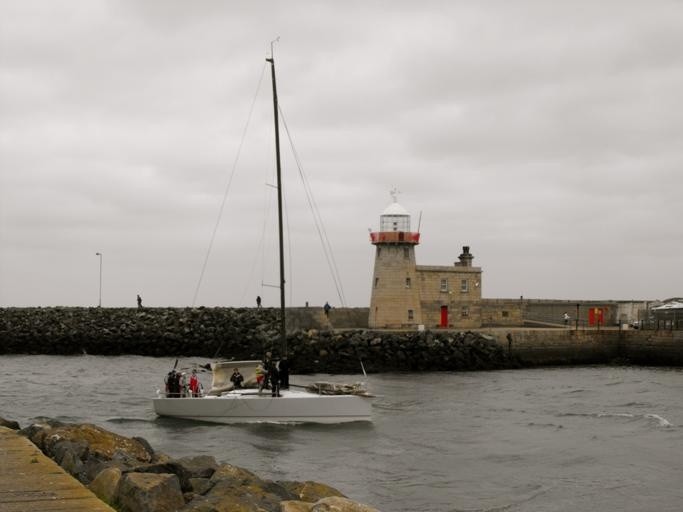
left=255, top=352, right=289, bottom=397
left=563, top=312, right=570, bottom=325
left=137, top=294, right=143, bottom=308
left=230, top=368, right=243, bottom=389
left=164, top=369, right=199, bottom=398
left=324, top=301, right=330, bottom=314
left=256, top=296, right=261, bottom=308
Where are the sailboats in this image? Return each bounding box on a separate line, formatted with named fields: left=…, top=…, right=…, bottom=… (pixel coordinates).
left=152, top=36, right=373, bottom=426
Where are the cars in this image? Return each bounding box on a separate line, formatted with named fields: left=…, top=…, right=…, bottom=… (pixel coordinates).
left=631, top=316, right=675, bottom=330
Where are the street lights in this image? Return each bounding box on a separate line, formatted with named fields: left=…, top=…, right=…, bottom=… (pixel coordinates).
left=95, top=252, right=103, bottom=307
